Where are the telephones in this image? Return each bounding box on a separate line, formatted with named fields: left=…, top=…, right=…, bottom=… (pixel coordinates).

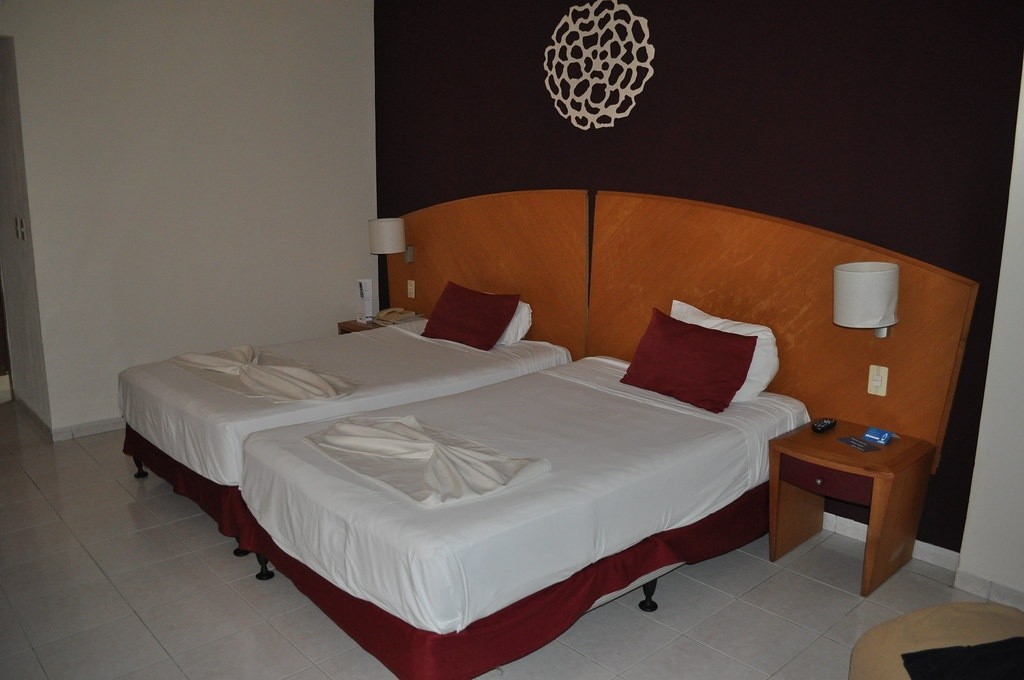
left=372, top=307, right=415, bottom=326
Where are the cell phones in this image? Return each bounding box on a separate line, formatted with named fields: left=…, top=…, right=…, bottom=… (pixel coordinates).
left=811, top=418, right=837, bottom=433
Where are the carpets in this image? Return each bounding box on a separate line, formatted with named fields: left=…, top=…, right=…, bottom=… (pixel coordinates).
left=850, top=605, right=1023, bottom=679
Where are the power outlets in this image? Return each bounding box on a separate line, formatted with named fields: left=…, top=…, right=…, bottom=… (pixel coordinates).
left=407, top=280, right=415, bottom=298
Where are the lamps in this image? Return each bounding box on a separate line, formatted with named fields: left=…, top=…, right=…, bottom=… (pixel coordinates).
left=367, top=218, right=413, bottom=263
left=833, top=262, right=899, bottom=338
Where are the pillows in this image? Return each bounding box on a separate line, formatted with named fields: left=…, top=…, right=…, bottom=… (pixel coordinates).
left=421, top=281, right=533, bottom=351
left=619, top=299, right=779, bottom=414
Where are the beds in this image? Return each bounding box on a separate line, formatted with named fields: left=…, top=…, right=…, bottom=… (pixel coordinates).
left=116, top=188, right=589, bottom=557
left=240, top=191, right=980, bottom=680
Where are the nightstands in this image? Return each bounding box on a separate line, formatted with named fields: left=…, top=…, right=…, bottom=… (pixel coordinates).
left=338, top=317, right=383, bottom=335
left=768, top=417, right=936, bottom=596
